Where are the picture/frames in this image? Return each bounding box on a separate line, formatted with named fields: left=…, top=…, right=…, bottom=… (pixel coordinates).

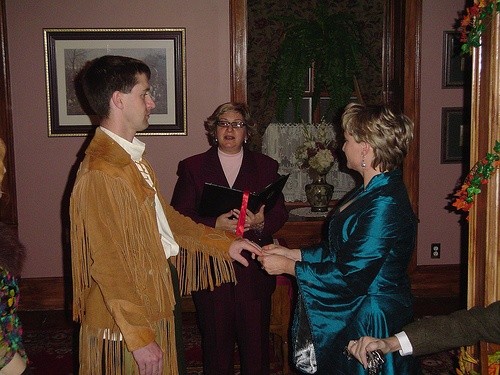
left=42, top=27, right=188, bottom=138
left=441, top=31, right=472, bottom=90
left=440, top=107, right=471, bottom=164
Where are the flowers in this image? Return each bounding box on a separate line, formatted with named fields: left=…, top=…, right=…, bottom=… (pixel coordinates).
left=452, top=139, right=500, bottom=222
left=295, top=115, right=339, bottom=173
left=458, top=0, right=500, bottom=55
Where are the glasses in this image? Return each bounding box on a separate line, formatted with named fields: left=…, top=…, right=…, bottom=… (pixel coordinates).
left=217, top=121, right=246, bottom=128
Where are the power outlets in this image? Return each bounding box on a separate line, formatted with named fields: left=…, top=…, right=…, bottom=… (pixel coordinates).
left=431, top=243, right=440, bottom=258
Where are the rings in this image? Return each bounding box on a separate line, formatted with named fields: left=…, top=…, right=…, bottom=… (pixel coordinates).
left=234, top=229, right=236, bottom=233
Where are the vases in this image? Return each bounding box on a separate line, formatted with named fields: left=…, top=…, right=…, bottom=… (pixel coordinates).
left=305, top=171, right=334, bottom=212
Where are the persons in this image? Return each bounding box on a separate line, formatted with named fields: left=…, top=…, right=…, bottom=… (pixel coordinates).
left=1, top=138, right=31, bottom=375
left=171, top=101, right=288, bottom=375
left=258, top=102, right=417, bottom=375
left=348, top=299, right=499, bottom=369
left=69, top=55, right=263, bottom=375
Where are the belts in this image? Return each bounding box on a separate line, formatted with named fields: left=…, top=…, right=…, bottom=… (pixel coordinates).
left=0, top=351, right=26, bottom=375
